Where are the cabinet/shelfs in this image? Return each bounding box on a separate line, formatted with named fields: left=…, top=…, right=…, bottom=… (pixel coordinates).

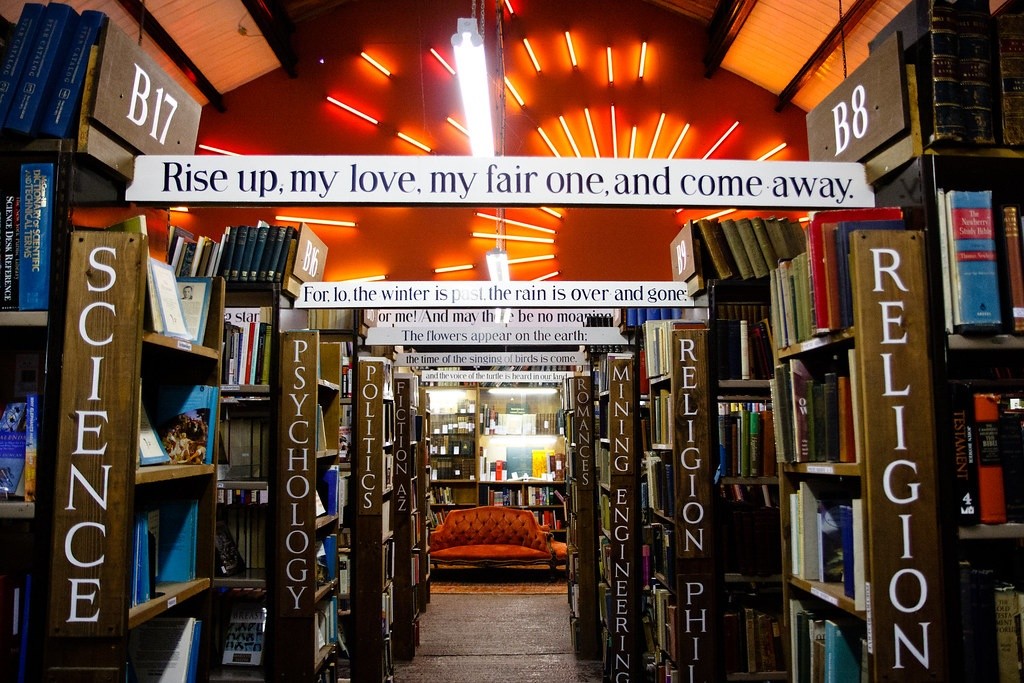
left=0, top=0, right=1024, bottom=683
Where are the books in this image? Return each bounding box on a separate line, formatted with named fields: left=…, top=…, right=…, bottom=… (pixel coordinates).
left=0, top=0, right=217, bottom=683
left=480, top=363, right=571, bottom=530
left=559, top=314, right=627, bottom=683
left=765, top=206, right=910, bottom=682
left=166, top=218, right=355, bottom=683
left=619, top=216, right=809, bottom=683
left=414, top=366, right=476, bottom=532
left=369, top=337, right=431, bottom=683
left=912, top=2, right=1024, bottom=682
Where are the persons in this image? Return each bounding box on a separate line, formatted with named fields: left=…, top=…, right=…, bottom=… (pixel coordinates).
left=180, top=285, right=194, bottom=300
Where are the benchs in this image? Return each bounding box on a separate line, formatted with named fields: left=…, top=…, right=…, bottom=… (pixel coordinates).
left=427, top=506, right=568, bottom=569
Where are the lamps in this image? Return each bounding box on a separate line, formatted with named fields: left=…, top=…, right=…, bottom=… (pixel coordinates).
left=450, top=0, right=494, bottom=154
left=484, top=208, right=512, bottom=322
